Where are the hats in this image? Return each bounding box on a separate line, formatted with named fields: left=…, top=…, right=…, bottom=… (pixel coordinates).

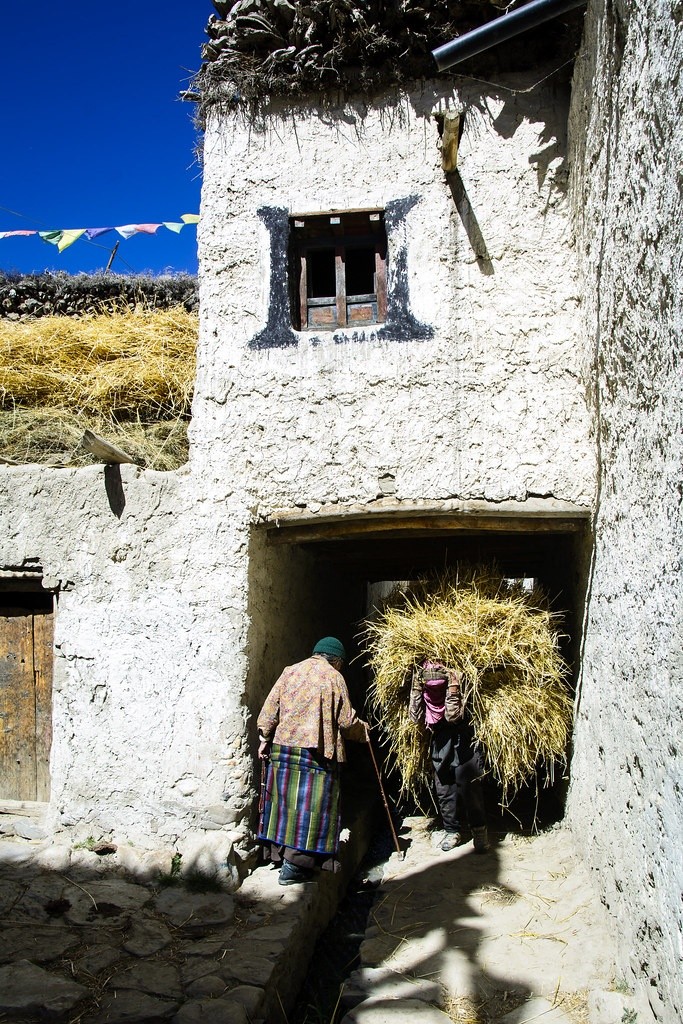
left=312, top=637, right=345, bottom=659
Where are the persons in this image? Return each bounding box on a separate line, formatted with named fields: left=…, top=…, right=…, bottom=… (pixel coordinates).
left=252, top=636, right=372, bottom=886
left=417, top=656, right=462, bottom=853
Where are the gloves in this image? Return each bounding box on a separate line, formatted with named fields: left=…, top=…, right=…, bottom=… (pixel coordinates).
left=447, top=670, right=463, bottom=687
left=413, top=673, right=422, bottom=691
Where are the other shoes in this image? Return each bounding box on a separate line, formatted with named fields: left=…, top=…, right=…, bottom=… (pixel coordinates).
left=278, top=866, right=309, bottom=884
left=471, top=828, right=488, bottom=852
left=441, top=832, right=469, bottom=851
